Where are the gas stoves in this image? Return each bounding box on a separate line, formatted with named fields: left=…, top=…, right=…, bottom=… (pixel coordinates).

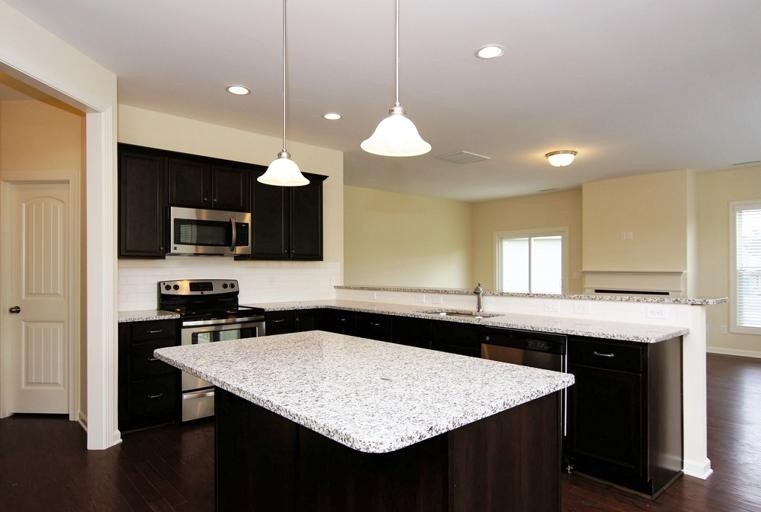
left=168, top=303, right=264, bottom=321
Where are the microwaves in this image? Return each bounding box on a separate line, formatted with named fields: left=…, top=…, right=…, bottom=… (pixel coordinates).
left=167, top=205, right=253, bottom=257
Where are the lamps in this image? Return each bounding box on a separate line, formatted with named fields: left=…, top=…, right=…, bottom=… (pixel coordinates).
left=256, top=0, right=311, bottom=189
left=545, top=150, right=578, bottom=167
left=361, top=0, right=431, bottom=158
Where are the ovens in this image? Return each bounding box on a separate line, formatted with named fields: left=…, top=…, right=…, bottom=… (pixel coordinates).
left=181, top=321, right=267, bottom=393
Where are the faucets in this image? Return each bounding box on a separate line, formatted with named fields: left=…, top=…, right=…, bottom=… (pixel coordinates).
left=472, top=282, right=484, bottom=313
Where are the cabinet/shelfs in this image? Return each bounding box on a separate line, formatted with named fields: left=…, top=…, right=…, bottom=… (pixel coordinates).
left=168, top=157, right=249, bottom=211
left=319, top=308, right=392, bottom=342
left=234, top=172, right=321, bottom=259
left=266, top=308, right=319, bottom=335
left=391, top=315, right=481, bottom=358
left=118, top=318, right=182, bottom=431
left=120, top=152, right=165, bottom=257
left=566, top=336, right=683, bottom=495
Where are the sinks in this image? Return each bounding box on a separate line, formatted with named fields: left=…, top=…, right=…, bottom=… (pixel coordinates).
left=425, top=310, right=505, bottom=318
